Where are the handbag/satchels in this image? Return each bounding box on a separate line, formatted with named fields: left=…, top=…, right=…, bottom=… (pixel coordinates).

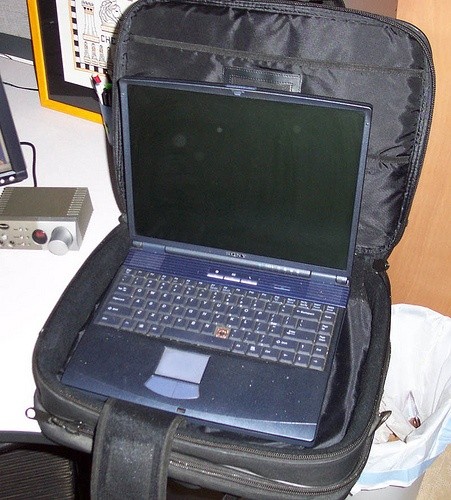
left=25, top=1, right=436, bottom=499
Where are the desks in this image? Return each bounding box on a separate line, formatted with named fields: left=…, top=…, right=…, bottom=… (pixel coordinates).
left=0, top=53, right=126, bottom=446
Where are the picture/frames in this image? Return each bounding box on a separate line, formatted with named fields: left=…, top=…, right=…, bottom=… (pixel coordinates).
left=25, top=0, right=137, bottom=123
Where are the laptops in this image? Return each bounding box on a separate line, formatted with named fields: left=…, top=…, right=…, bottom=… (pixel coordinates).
left=57, top=77, right=375, bottom=451
